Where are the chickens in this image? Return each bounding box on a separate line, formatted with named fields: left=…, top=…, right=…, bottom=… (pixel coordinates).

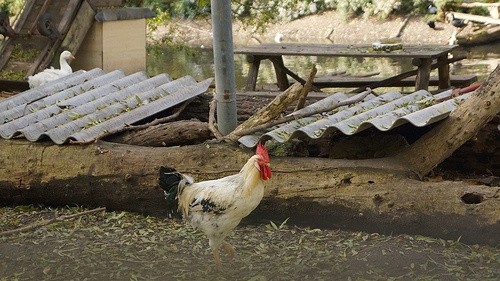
left=158, top=141, right=272, bottom=272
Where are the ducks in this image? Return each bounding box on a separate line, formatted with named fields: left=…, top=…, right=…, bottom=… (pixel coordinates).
left=451, top=13, right=465, bottom=28
left=28, top=50, right=75, bottom=89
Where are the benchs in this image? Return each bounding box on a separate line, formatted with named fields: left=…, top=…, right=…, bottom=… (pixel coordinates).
left=275, top=74, right=480, bottom=90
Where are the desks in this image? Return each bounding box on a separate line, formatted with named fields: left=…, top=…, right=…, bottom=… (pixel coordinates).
left=232, top=41, right=460, bottom=94
